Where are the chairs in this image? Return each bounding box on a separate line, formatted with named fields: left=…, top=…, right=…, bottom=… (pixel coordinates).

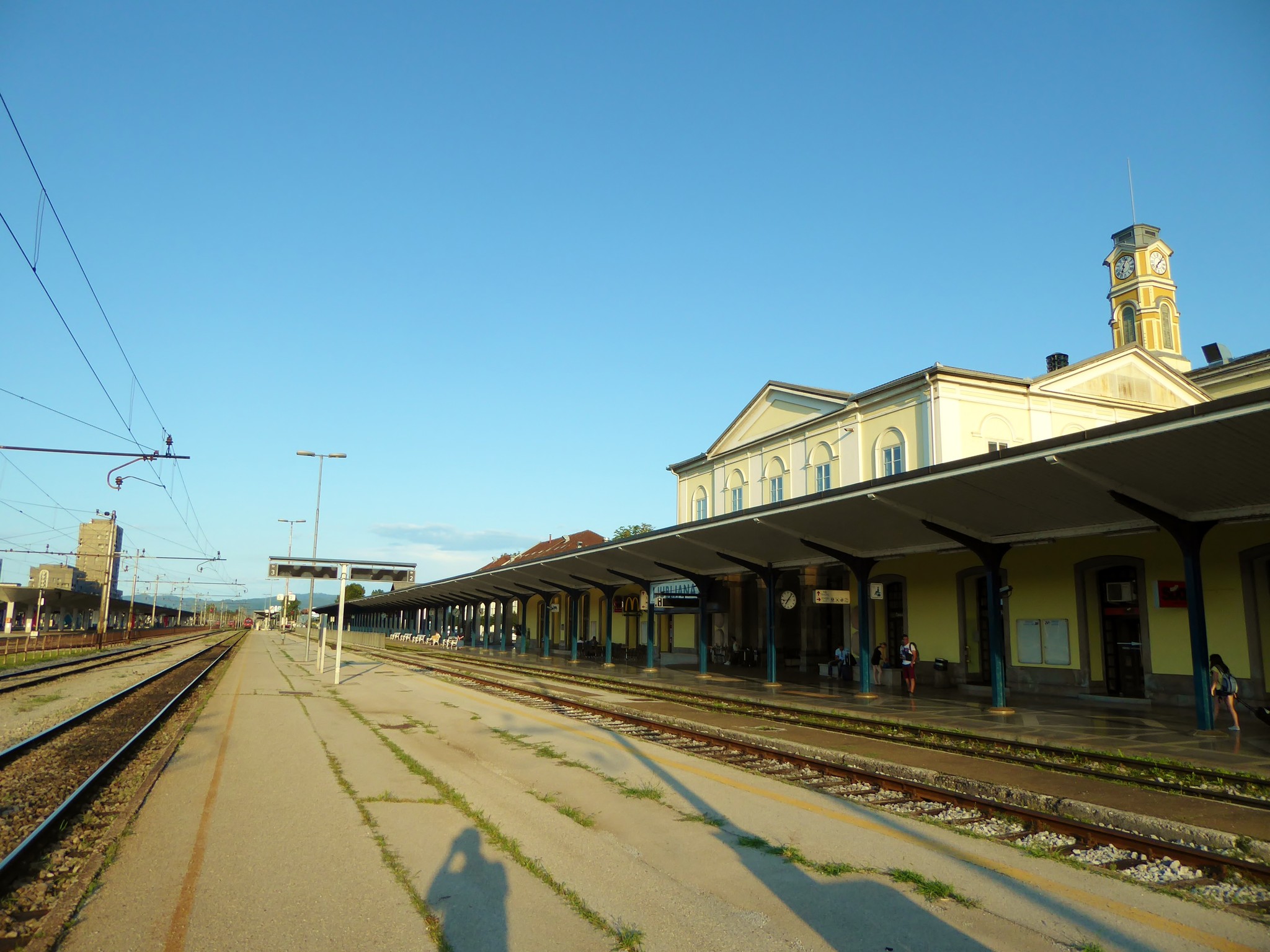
left=707, top=646, right=758, bottom=667
left=577, top=642, right=645, bottom=661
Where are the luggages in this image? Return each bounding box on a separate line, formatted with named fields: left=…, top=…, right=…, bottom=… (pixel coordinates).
left=1234, top=697, right=1270, bottom=725
left=841, top=650, right=853, bottom=681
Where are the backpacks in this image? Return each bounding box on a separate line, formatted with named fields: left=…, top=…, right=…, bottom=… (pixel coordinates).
left=901, top=642, right=920, bottom=663
left=1210, top=664, right=1238, bottom=696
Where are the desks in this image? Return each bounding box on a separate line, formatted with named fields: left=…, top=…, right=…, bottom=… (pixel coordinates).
left=620, top=646, right=630, bottom=660
left=592, top=645, right=606, bottom=657
left=739, top=648, right=753, bottom=667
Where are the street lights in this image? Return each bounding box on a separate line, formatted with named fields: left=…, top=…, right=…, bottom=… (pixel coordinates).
left=265, top=578, right=280, bottom=631
left=297, top=450, right=347, bottom=662
left=277, top=518, right=307, bottom=633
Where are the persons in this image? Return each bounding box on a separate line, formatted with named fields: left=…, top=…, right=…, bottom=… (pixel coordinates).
left=724, top=636, right=738, bottom=665
left=577, top=636, right=597, bottom=658
left=827, top=643, right=850, bottom=680
left=900, top=634, right=917, bottom=696
left=1209, top=654, right=1240, bottom=730
left=435, top=631, right=440, bottom=645
left=871, top=642, right=887, bottom=686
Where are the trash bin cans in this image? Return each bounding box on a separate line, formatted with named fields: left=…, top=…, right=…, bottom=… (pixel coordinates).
left=934, top=658, right=949, bottom=688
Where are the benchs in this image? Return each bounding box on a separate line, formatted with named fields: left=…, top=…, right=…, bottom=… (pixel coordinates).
left=390, top=632, right=401, bottom=640
left=426, top=636, right=440, bottom=646
left=442, top=636, right=458, bottom=647
left=817, top=663, right=902, bottom=687
left=399, top=633, right=412, bottom=641
left=412, top=634, right=426, bottom=643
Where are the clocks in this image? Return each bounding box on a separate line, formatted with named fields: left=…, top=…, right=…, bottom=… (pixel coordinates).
left=1114, top=257, right=1134, bottom=280
left=780, top=591, right=797, bottom=609
left=1150, top=251, right=1166, bottom=274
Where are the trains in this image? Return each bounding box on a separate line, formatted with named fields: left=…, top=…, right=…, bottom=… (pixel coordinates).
left=277, top=621, right=292, bottom=629
left=243, top=616, right=254, bottom=629
left=228, top=620, right=235, bottom=626
left=215, top=621, right=220, bottom=625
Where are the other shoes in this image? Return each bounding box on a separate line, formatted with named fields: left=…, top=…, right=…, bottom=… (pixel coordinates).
left=827, top=677, right=833, bottom=679
left=904, top=692, right=914, bottom=699
left=1228, top=726, right=1240, bottom=730
left=724, top=661, right=729, bottom=665
left=835, top=678, right=841, bottom=680
left=874, top=682, right=886, bottom=686
